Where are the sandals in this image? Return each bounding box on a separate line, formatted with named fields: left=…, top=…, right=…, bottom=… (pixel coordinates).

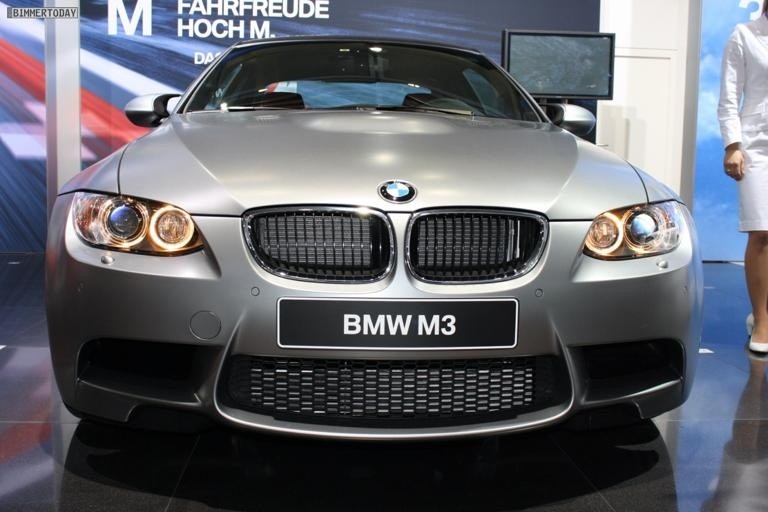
left=747, top=323, right=768, bottom=354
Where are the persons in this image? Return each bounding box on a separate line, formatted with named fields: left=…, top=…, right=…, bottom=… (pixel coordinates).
left=716, top=0, right=768, bottom=357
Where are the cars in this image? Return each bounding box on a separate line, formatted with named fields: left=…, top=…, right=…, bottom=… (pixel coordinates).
left=38, top=34, right=707, bottom=445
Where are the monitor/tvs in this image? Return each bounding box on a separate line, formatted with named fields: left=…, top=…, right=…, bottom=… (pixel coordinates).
left=502, top=28, right=615, bottom=99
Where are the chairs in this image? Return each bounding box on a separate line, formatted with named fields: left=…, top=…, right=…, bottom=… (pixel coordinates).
left=210, top=90, right=306, bottom=111
left=400, top=91, right=481, bottom=113
